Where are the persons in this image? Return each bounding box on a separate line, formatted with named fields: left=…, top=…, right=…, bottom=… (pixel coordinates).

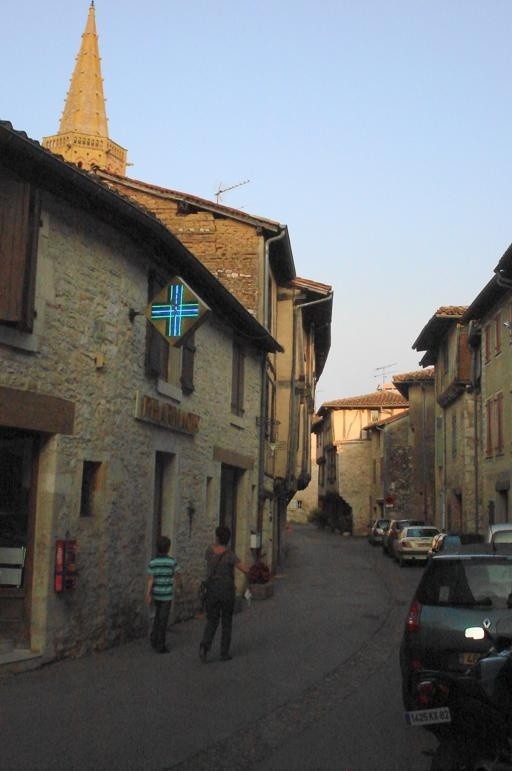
left=144, top=536, right=184, bottom=654
left=198, top=525, right=249, bottom=664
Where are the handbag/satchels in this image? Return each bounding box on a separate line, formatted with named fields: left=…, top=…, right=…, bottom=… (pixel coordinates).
left=198, top=580, right=210, bottom=605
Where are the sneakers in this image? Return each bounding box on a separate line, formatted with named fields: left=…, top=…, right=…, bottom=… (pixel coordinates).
left=198, top=645, right=208, bottom=664
left=219, top=653, right=232, bottom=661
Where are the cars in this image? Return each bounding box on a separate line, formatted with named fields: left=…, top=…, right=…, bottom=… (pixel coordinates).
left=369, top=519, right=512, bottom=711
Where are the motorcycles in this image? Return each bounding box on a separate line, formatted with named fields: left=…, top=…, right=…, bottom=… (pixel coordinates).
left=404, top=627, right=512, bottom=771
